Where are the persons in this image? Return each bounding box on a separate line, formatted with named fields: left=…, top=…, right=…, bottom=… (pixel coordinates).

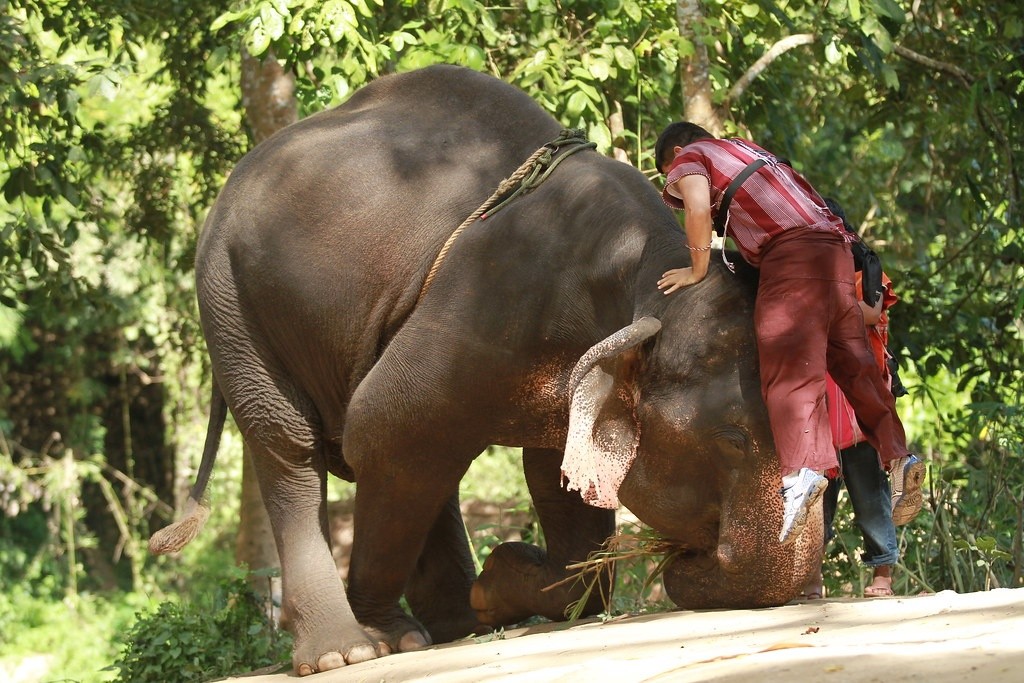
left=796, top=197, right=899, bottom=600
left=655, top=123, right=926, bottom=544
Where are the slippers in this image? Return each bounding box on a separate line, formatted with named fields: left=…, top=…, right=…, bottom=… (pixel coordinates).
left=799, top=586, right=822, bottom=600
left=864, top=574, right=894, bottom=597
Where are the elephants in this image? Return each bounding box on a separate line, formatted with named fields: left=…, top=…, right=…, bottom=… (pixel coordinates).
left=146, top=64, right=829, bottom=675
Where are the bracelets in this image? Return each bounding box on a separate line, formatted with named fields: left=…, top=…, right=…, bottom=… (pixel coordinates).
left=685, top=240, right=714, bottom=251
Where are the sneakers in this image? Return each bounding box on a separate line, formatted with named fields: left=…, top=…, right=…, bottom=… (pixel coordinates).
left=890, top=453, right=926, bottom=526
left=777, top=466, right=829, bottom=546
